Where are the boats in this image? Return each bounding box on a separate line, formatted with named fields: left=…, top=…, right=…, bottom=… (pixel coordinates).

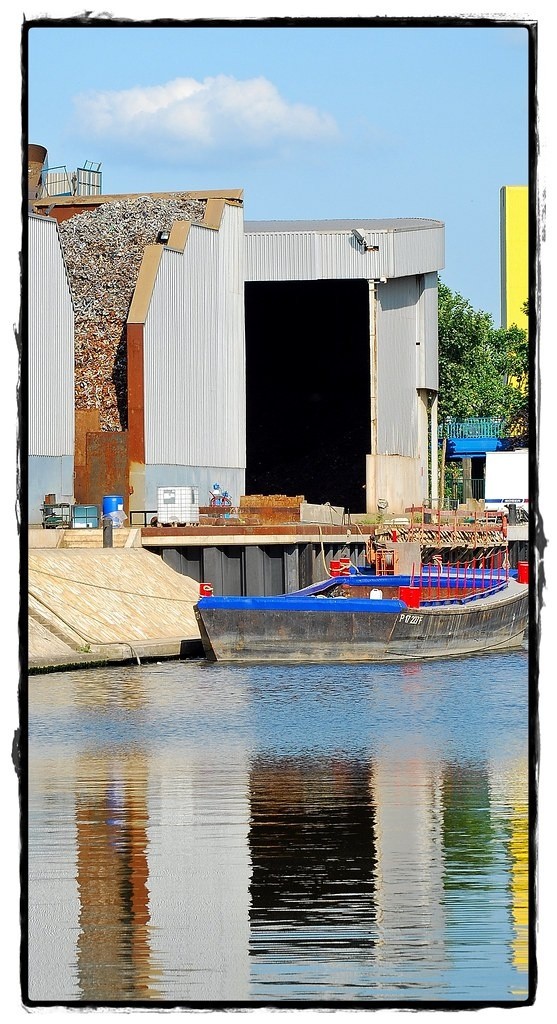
left=193, top=547, right=530, bottom=662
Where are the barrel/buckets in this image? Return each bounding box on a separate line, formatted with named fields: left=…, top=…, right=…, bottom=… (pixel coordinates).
left=103, top=495, right=124, bottom=528
left=370, top=589, right=383, bottom=599
